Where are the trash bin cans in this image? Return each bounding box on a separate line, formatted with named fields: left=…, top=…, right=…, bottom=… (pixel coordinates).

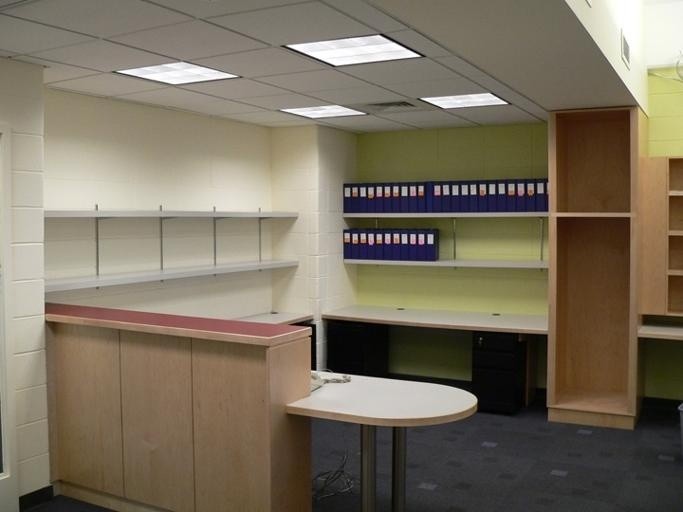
left=678, top=404, right=683, bottom=457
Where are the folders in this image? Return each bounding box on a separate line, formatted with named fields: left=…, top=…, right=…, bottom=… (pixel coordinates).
left=344, top=179, right=547, bottom=213
left=344, top=228, right=440, bottom=261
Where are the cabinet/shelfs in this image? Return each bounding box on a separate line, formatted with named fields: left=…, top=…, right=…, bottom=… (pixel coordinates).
left=665, top=157, right=683, bottom=317
left=42, top=300, right=314, bottom=511
left=42, top=209, right=299, bottom=294
left=550, top=108, right=637, bottom=432
left=340, top=211, right=550, bottom=270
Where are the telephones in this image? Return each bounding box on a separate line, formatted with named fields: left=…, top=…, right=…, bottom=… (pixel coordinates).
left=311, top=372, right=322, bottom=391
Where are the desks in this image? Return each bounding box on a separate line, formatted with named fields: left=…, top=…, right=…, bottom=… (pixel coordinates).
left=636, top=322, right=682, bottom=341
left=321, top=303, right=548, bottom=419
left=284, top=368, right=479, bottom=511
left=230, top=310, right=315, bottom=372
